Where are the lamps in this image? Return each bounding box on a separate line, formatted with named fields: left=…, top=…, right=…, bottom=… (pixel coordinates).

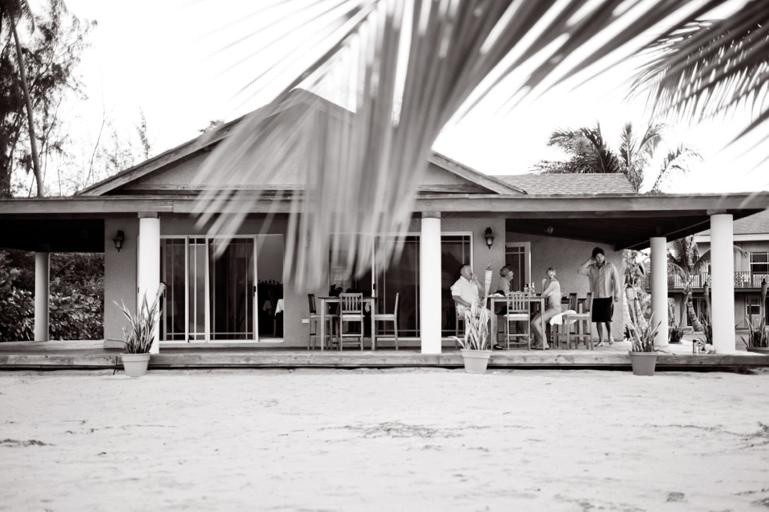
left=111, top=230, right=125, bottom=252
left=484, top=226, right=494, bottom=248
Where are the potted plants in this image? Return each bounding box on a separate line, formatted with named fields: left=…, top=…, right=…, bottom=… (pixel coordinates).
left=739, top=314, right=769, bottom=354
left=111, top=284, right=166, bottom=377
left=447, top=300, right=492, bottom=373
left=624, top=310, right=662, bottom=375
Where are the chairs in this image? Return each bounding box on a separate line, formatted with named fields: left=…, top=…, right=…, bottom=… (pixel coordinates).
left=306, top=292, right=401, bottom=353
left=454, top=291, right=596, bottom=351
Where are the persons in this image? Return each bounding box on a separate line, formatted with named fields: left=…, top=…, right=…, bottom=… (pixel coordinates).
left=578, top=247, right=621, bottom=348
left=493, top=265, right=532, bottom=344
left=528, top=268, right=563, bottom=350
left=449, top=265, right=504, bottom=351
left=329, top=280, right=372, bottom=336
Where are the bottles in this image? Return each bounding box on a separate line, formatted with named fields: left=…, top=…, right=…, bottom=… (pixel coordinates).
left=531, top=281, right=537, bottom=297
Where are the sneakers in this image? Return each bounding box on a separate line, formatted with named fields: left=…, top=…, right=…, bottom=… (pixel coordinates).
left=489, top=343, right=503, bottom=350
left=532, top=344, right=549, bottom=349
